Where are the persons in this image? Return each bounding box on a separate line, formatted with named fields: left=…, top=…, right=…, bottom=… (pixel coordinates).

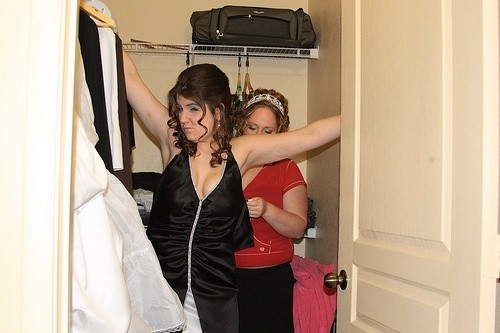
left=122, top=46, right=341, bottom=333
left=234, top=87, right=309, bottom=333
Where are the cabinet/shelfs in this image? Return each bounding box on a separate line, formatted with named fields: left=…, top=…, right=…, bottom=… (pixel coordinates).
left=121, top=42, right=320, bottom=244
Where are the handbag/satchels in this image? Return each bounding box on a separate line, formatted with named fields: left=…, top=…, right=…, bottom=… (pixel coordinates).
left=190, top=6, right=316, bottom=54
